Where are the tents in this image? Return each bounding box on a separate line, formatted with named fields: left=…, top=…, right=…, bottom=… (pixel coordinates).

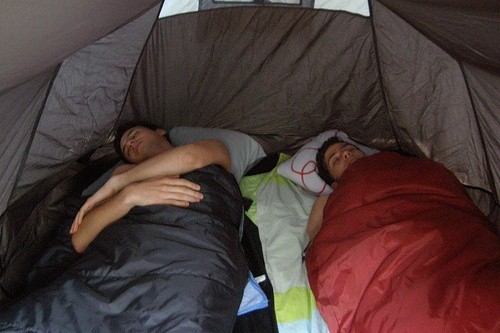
left=0, top=0, right=500, bottom=229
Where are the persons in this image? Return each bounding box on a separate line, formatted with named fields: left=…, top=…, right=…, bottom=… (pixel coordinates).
left=307, top=134, right=368, bottom=241
left=70, top=121, right=234, bottom=254
